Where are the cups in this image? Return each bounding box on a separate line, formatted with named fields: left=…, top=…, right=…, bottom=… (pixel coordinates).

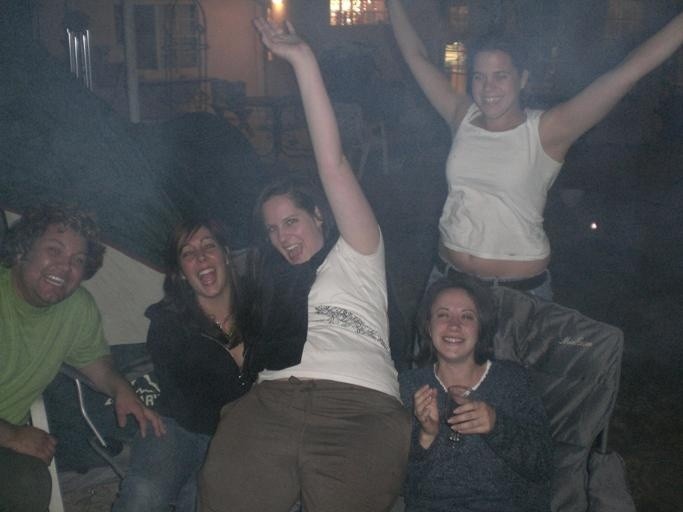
left=440, top=383, right=475, bottom=444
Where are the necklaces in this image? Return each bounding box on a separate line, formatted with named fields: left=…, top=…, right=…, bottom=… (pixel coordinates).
left=213, top=312, right=232, bottom=329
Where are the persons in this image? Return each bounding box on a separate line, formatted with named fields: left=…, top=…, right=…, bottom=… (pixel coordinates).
left=191, top=9, right=411, bottom=511
left=382, top=0, right=682, bottom=309
left=140, top=212, right=249, bottom=434
left=393, top=276, right=556, bottom=510
left=0, top=200, right=167, bottom=511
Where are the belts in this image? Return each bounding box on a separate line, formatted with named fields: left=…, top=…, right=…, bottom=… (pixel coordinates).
left=434, top=255, right=546, bottom=290
